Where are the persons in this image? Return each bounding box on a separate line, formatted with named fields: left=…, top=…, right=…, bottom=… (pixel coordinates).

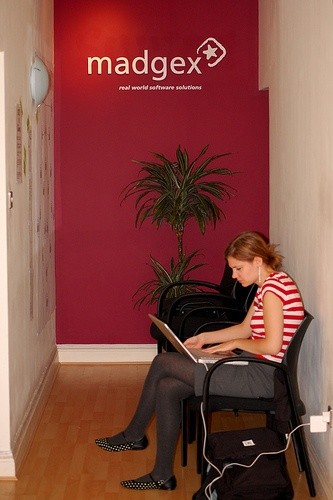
left=95, top=231, right=306, bottom=491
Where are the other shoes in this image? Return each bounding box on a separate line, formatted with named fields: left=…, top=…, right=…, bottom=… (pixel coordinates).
left=121, top=473, right=176, bottom=490
left=95, top=432, right=148, bottom=452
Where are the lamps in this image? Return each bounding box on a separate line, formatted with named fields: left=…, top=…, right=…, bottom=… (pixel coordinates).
left=30, top=55, right=50, bottom=108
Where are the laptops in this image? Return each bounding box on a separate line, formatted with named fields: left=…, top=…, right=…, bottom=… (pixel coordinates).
left=148, top=314, right=249, bottom=365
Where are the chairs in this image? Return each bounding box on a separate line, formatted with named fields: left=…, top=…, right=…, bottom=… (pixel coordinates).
left=150, top=260, right=317, bottom=497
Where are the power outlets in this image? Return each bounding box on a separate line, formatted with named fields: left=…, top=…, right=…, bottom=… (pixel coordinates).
left=8, top=191, right=14, bottom=209
left=326, top=406, right=333, bottom=427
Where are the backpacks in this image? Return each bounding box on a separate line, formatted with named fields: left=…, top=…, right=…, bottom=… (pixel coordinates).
left=192, top=428, right=294, bottom=500
